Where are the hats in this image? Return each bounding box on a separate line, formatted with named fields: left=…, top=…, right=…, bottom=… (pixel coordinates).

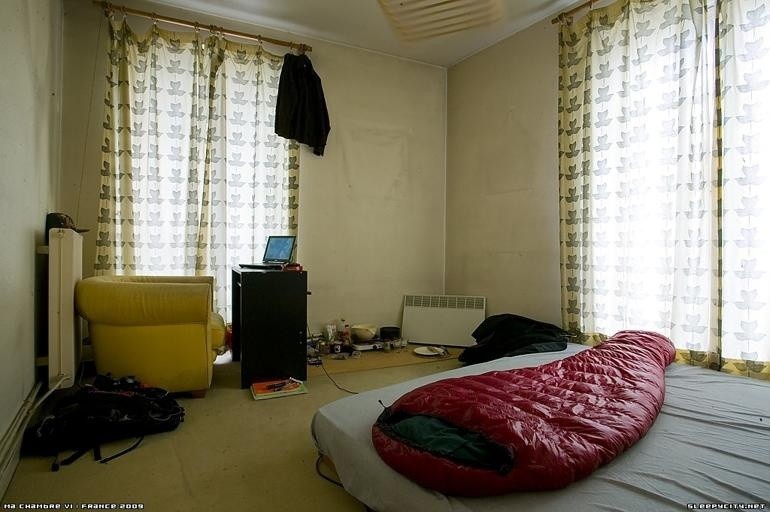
left=45, top=212, right=91, bottom=241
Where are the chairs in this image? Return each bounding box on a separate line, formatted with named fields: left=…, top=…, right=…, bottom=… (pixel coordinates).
left=76, top=275, right=227, bottom=397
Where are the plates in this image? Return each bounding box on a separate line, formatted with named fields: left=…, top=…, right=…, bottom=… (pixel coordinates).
left=414, top=346, right=445, bottom=356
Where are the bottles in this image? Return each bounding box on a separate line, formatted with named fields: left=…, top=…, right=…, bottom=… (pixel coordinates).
left=343, top=324, right=351, bottom=346
left=384, top=339, right=391, bottom=353
left=337, top=318, right=346, bottom=341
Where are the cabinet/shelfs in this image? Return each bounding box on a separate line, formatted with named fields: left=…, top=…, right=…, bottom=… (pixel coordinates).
left=231, top=265, right=308, bottom=389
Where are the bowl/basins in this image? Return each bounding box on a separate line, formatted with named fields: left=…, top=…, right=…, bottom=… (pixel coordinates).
left=352, top=323, right=379, bottom=339
left=380, top=326, right=400, bottom=340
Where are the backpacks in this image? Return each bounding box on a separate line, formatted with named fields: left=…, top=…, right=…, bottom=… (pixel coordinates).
left=17, top=371, right=186, bottom=461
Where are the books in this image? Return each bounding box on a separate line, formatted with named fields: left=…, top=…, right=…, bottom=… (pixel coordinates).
left=250, top=380, right=309, bottom=401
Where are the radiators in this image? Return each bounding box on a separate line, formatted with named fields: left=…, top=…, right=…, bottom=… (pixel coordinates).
left=37, top=229, right=82, bottom=389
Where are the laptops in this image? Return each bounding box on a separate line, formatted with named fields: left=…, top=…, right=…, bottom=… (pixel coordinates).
left=238, top=235, right=296, bottom=269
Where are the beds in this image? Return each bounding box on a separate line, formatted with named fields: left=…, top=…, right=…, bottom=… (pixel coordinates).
left=311, top=340, right=770, bottom=510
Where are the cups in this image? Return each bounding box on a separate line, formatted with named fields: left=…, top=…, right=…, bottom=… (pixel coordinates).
left=324, top=323, right=337, bottom=341
left=393, top=336, right=402, bottom=354
